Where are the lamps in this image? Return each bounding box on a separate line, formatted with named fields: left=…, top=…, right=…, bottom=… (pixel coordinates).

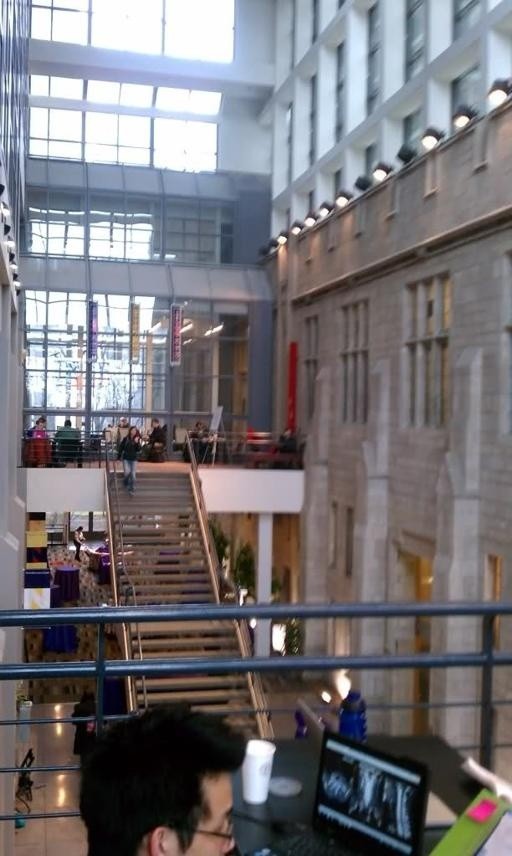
left=421, top=127, right=446, bottom=149
left=256, top=220, right=304, bottom=255
left=319, top=202, right=333, bottom=217
left=451, top=105, right=474, bottom=129
left=306, top=212, right=319, bottom=228
left=336, top=191, right=353, bottom=208
left=487, top=78, right=509, bottom=107
left=373, top=162, right=392, bottom=182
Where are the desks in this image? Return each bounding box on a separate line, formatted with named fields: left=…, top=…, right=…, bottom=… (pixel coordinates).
left=45, top=565, right=80, bottom=650
left=228, top=731, right=494, bottom=855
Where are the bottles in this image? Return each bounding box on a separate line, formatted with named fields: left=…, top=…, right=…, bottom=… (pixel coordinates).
left=340, top=690, right=367, bottom=742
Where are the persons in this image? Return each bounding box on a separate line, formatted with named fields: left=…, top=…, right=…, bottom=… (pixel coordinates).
left=74, top=525, right=86, bottom=562
left=80, top=698, right=248, bottom=856
left=71, top=692, right=98, bottom=767
left=117, top=424, right=147, bottom=495
left=26, top=415, right=298, bottom=469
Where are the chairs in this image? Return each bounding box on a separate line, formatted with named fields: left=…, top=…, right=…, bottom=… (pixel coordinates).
left=24, top=427, right=304, bottom=469
left=86, top=536, right=141, bottom=583
left=14, top=749, right=37, bottom=812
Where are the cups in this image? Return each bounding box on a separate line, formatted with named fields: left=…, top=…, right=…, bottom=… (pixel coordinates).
left=242, top=739, right=277, bottom=805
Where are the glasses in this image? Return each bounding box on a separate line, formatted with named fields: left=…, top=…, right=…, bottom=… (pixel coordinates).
left=168, top=821, right=235, bottom=842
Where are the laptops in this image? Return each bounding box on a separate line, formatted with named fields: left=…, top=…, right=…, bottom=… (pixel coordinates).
left=242, top=728, right=431, bottom=856
